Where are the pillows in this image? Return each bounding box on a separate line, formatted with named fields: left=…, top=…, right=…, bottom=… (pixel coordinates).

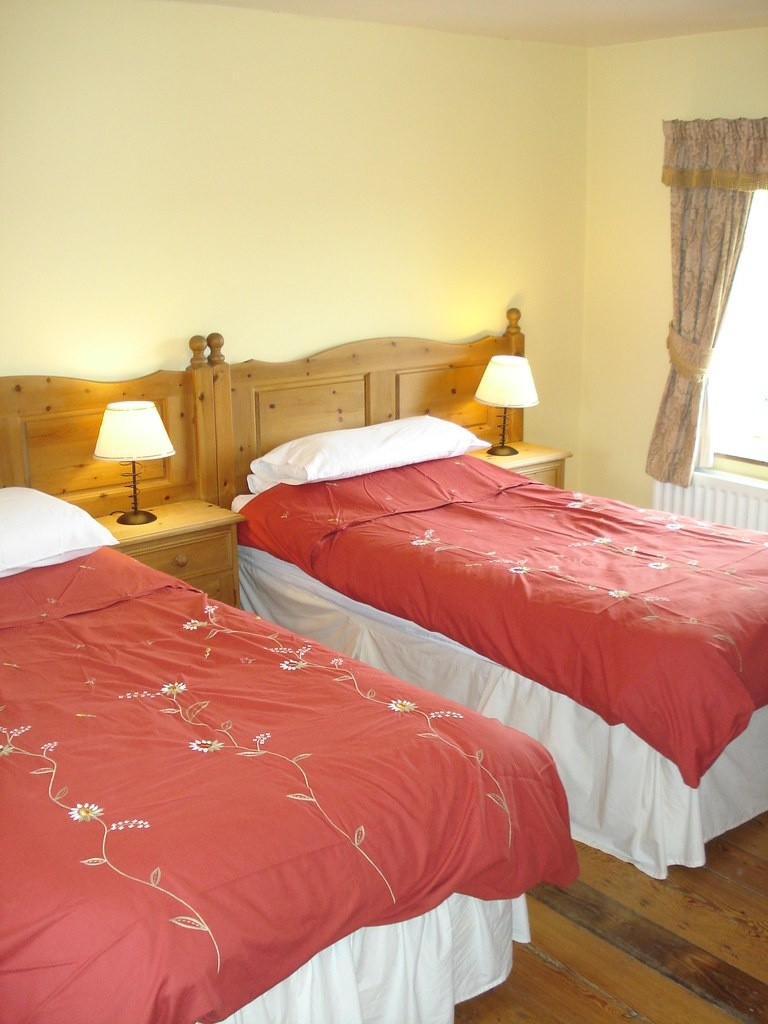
left=0, top=486, right=120, bottom=579
left=248, top=415, right=492, bottom=486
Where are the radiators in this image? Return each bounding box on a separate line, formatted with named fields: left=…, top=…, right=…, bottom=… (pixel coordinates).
left=652, top=468, right=768, bottom=532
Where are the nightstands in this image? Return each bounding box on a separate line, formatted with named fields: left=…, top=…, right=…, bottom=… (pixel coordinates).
left=469, top=441, right=573, bottom=490
left=97, top=499, right=246, bottom=607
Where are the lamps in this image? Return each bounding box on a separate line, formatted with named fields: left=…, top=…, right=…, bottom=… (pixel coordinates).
left=93, top=401, right=177, bottom=525
left=474, top=355, right=539, bottom=456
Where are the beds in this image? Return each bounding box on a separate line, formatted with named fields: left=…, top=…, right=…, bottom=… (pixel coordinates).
left=207, top=307, right=768, bottom=879
left=0, top=336, right=579, bottom=1024
